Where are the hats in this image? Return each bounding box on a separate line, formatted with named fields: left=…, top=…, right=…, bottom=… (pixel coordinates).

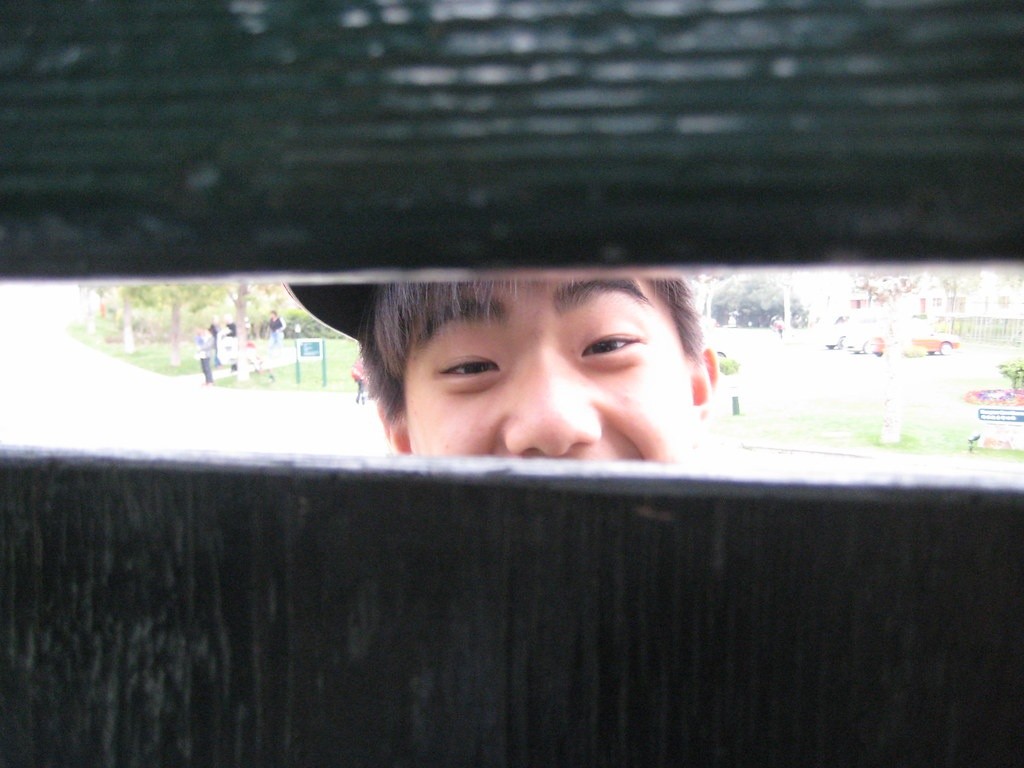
left=285, top=283, right=370, bottom=341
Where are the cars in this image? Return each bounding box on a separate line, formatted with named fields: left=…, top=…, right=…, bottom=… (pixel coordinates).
left=822, top=307, right=960, bottom=358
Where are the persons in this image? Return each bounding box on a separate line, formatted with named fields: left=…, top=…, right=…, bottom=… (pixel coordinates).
left=264, top=310, right=287, bottom=371
left=195, top=314, right=237, bottom=385
left=284, top=273, right=720, bottom=465
